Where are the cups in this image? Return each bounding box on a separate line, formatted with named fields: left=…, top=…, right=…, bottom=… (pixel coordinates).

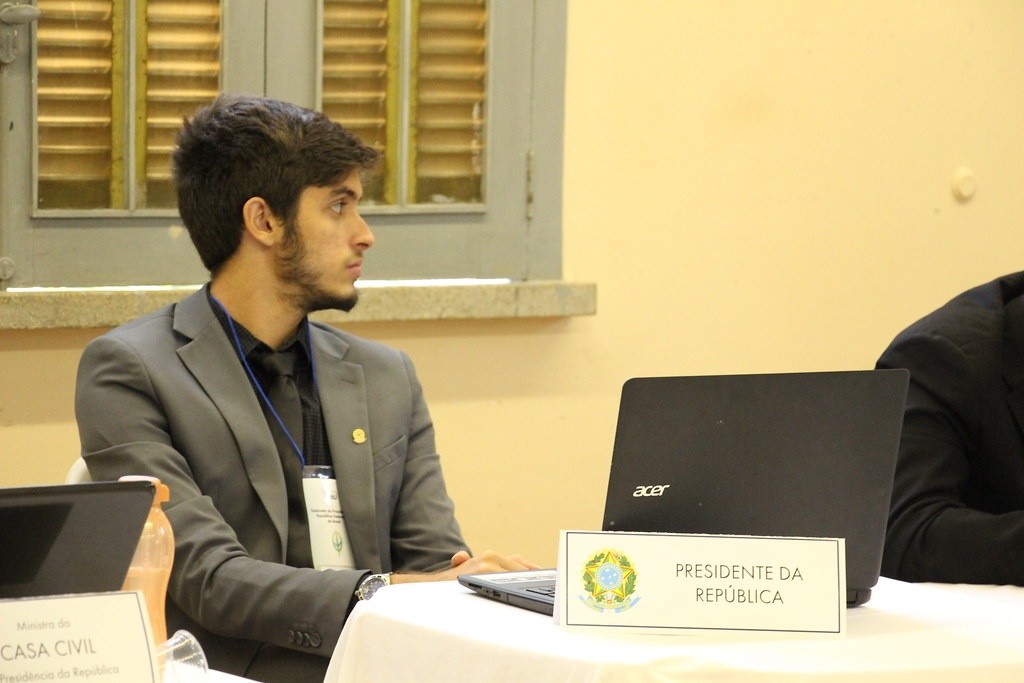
left=153, top=629, right=209, bottom=683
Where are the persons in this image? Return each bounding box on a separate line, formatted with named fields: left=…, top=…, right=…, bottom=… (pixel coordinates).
left=871, top=270, right=1024, bottom=593
left=72, top=90, right=547, bottom=683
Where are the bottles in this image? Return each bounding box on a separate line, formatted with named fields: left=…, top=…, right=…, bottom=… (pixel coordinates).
left=116, top=475, right=176, bottom=683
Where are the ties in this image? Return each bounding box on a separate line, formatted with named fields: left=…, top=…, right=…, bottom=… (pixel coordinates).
left=248, top=346, right=315, bottom=570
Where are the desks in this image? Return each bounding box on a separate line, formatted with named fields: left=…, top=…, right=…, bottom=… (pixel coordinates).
left=318, top=567, right=1024, bottom=683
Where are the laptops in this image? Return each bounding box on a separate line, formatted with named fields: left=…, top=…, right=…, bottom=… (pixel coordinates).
left=0, top=482, right=157, bottom=598
left=458, top=369, right=911, bottom=617
